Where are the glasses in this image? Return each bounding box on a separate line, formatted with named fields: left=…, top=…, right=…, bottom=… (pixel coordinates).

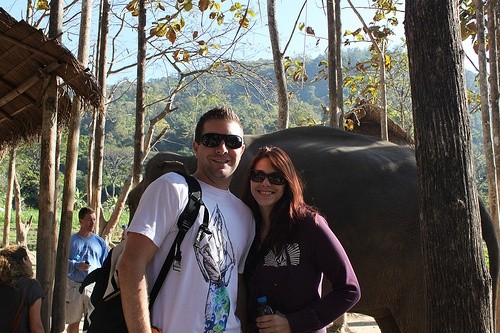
left=247, top=170, right=287, bottom=185
left=195, top=132, right=243, bottom=149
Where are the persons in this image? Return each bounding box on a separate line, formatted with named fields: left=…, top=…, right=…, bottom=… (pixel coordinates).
left=240, top=146, right=361, bottom=333
left=0, top=245, right=48, bottom=333
left=64, top=207, right=111, bottom=333
left=115, top=105, right=258, bottom=333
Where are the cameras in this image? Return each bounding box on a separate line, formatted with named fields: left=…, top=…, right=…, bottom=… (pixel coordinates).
left=258, top=304, right=277, bottom=317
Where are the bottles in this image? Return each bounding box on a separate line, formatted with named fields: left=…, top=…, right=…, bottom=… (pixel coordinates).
left=256, top=295, right=275, bottom=318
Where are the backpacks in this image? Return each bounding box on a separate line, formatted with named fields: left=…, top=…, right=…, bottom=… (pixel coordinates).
left=78, top=168, right=212, bottom=333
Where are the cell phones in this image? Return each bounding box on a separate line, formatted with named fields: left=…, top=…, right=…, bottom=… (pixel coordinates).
left=85, top=260, right=89, bottom=265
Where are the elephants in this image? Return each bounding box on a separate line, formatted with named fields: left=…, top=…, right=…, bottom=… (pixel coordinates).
left=127, top=125, right=500, bottom=333
